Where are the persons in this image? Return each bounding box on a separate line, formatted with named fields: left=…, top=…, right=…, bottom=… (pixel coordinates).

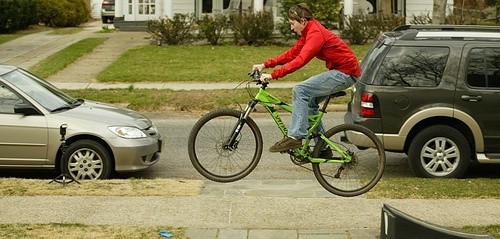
left=252, top=3, right=361, bottom=152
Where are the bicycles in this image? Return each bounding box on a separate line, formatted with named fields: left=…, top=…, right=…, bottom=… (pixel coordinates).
left=187, top=67, right=386, bottom=197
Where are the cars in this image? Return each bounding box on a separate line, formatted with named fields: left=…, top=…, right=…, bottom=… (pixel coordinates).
left=101, top=0, right=116, bottom=24
left=0, top=64, right=163, bottom=185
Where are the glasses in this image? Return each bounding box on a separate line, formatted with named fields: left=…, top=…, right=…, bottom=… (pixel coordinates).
left=288, top=7, right=302, bottom=19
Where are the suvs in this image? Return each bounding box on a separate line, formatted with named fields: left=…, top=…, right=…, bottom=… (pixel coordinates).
left=340, top=23, right=500, bottom=179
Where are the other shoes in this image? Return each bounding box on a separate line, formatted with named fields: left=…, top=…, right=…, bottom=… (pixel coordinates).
left=269, top=137, right=303, bottom=153
left=304, top=146, right=333, bottom=163
